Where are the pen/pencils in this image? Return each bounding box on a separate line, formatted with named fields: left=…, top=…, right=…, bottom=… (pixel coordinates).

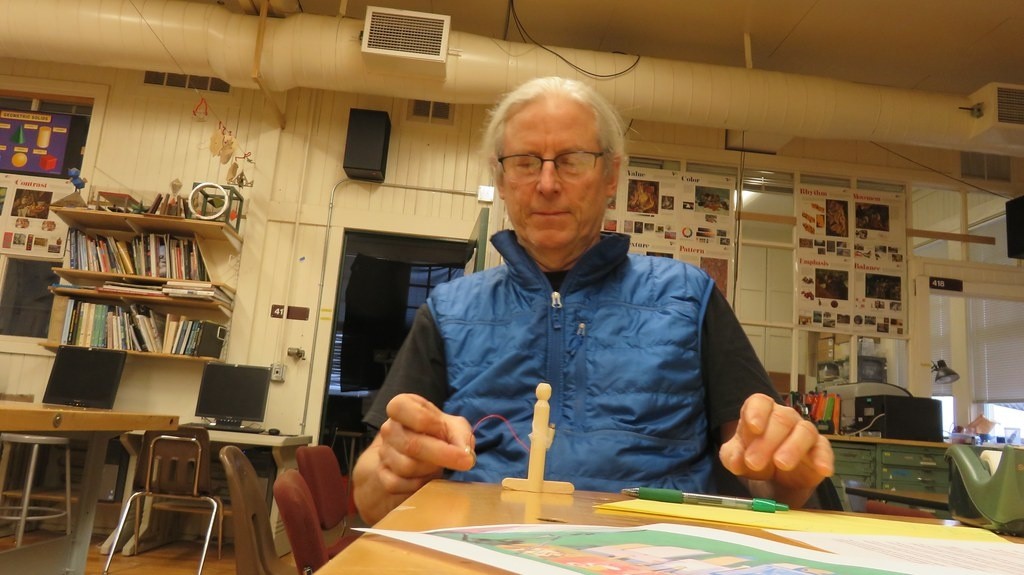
left=622, top=488, right=790, bottom=513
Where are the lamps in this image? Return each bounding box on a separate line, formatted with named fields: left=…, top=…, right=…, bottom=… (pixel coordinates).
left=929, top=360, right=960, bottom=384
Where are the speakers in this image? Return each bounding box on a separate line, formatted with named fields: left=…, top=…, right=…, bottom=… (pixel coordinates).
left=343, top=108, right=391, bottom=181
left=1005, top=195, right=1024, bottom=259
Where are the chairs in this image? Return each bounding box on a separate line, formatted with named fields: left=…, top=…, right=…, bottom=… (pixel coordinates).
left=104, top=423, right=385, bottom=575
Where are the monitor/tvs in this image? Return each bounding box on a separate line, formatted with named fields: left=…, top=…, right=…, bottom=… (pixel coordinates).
left=194, top=361, right=272, bottom=427
left=43, top=345, right=128, bottom=409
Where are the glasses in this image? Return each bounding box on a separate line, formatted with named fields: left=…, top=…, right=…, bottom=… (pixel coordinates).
left=497, top=150, right=603, bottom=185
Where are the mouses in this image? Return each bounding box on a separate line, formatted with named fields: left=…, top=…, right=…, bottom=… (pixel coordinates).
left=269, top=429, right=280, bottom=435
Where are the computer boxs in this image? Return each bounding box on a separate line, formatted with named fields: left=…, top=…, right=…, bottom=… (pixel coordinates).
left=247, top=448, right=277, bottom=518
left=96, top=438, right=131, bottom=502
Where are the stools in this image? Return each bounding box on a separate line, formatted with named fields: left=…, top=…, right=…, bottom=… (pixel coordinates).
left=0, top=431, right=74, bottom=557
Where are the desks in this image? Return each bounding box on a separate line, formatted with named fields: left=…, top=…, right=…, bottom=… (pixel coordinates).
left=305, top=484, right=1022, bottom=575
left=123, top=429, right=312, bottom=558
left=0, top=400, right=175, bottom=538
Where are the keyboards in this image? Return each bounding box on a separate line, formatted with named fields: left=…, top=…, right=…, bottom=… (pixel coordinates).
left=187, top=422, right=266, bottom=434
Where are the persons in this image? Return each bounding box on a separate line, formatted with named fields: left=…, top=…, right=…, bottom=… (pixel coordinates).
left=350, top=78, right=835, bottom=530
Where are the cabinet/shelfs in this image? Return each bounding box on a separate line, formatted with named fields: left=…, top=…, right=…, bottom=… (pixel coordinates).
left=36, top=206, right=245, bottom=366
left=824, top=441, right=970, bottom=510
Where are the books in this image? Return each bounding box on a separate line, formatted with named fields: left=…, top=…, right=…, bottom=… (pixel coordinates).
left=69, top=227, right=205, bottom=280
left=53, top=279, right=234, bottom=305
left=60, top=299, right=229, bottom=357
left=807, top=391, right=840, bottom=433
